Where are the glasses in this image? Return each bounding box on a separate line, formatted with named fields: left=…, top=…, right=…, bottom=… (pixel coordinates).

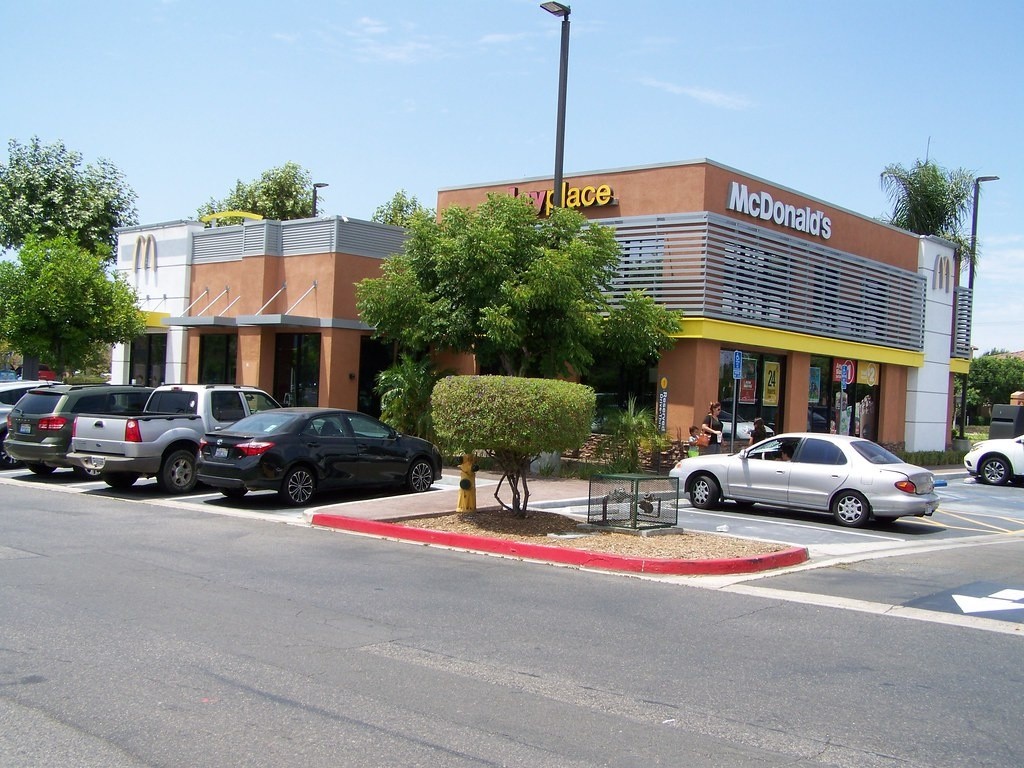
left=714, top=408, right=721, bottom=411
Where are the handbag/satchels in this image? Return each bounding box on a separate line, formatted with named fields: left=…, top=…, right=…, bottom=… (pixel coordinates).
left=696, top=433, right=711, bottom=448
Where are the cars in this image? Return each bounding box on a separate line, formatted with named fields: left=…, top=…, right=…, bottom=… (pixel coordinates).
left=15, top=363, right=57, bottom=382
left=193, top=407, right=442, bottom=507
left=0, top=380, right=61, bottom=467
left=667, top=431, right=942, bottom=528
left=591, top=392, right=627, bottom=432
left=962, top=434, right=1024, bottom=487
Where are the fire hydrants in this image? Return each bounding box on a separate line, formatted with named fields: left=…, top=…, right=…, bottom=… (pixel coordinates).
left=457, top=449, right=481, bottom=514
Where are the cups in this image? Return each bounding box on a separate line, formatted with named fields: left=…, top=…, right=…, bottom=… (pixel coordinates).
left=860, top=414, right=875, bottom=440
left=717, top=433, right=722, bottom=443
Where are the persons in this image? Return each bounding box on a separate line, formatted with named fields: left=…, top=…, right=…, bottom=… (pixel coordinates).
left=749, top=417, right=765, bottom=445
left=701, top=402, right=724, bottom=455
left=688, top=426, right=701, bottom=458
left=774, top=446, right=794, bottom=462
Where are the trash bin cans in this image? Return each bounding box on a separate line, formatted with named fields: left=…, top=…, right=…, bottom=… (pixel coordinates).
left=988, top=405, right=1024, bottom=440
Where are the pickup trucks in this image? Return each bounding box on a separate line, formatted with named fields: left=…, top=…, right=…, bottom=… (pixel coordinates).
left=64, top=381, right=287, bottom=496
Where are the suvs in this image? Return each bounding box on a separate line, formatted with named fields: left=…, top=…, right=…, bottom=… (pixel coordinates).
left=3, top=381, right=159, bottom=477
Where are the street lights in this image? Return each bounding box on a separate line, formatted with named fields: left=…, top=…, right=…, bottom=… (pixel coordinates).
left=540, top=0, right=572, bottom=214
left=961, top=176, right=996, bottom=441
left=311, top=182, right=330, bottom=217
